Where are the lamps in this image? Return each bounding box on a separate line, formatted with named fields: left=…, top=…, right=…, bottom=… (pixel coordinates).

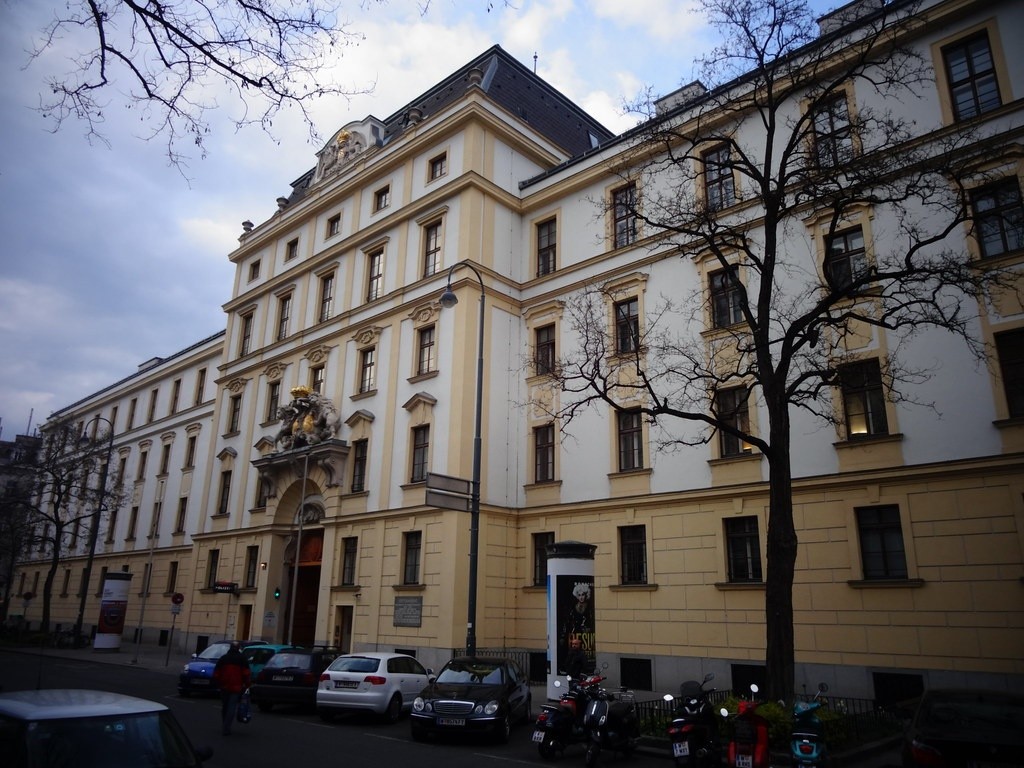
left=261, top=562, right=267, bottom=570
left=274, top=587, right=281, bottom=599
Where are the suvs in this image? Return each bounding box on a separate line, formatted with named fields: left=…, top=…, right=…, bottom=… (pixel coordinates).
left=256, top=644, right=350, bottom=711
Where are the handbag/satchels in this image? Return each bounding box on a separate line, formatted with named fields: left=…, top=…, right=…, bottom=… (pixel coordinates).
left=236, top=694, right=252, bottom=724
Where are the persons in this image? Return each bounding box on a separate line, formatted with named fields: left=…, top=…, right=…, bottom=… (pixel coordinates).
left=560, top=583, right=592, bottom=642
left=214, top=641, right=251, bottom=736
left=566, top=640, right=587, bottom=678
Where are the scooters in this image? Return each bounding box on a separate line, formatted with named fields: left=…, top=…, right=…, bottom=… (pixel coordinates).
left=778, top=682, right=830, bottom=766
left=719, top=681, right=776, bottom=768
left=531, top=661, right=642, bottom=766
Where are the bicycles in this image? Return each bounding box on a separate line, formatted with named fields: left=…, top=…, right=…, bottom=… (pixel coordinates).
left=51, top=627, right=91, bottom=649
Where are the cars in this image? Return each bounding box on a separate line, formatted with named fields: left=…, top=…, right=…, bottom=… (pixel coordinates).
left=895, top=686, right=1024, bottom=768
left=240, top=645, right=303, bottom=690
left=316, top=652, right=434, bottom=726
left=0, top=688, right=213, bottom=768
left=411, top=656, right=532, bottom=743
left=180, top=640, right=268, bottom=695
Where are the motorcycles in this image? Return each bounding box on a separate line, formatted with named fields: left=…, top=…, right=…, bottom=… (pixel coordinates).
left=663, top=672, right=721, bottom=768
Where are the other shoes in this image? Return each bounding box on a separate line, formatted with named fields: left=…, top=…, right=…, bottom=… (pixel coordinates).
left=223, top=732, right=232, bottom=736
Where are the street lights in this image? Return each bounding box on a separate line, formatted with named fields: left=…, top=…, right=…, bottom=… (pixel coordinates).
left=74, top=417, right=114, bottom=649
left=436, top=262, right=485, bottom=648
